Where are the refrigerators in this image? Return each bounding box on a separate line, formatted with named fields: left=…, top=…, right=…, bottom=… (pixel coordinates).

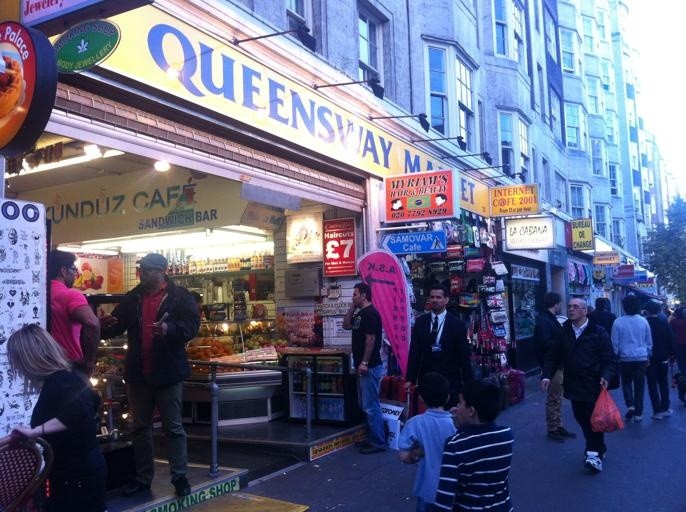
left=281, top=352, right=366, bottom=428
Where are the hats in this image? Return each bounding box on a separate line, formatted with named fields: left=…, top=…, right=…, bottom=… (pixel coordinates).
left=136, top=253, right=167, bottom=271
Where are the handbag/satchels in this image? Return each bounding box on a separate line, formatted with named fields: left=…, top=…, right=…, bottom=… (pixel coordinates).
left=407, top=221, right=508, bottom=367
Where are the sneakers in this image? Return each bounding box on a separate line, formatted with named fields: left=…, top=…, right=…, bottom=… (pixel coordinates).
left=354, top=441, right=385, bottom=454
left=174, top=476, right=190, bottom=497
left=624, top=406, right=674, bottom=421
left=548, top=427, right=576, bottom=441
left=582, top=451, right=605, bottom=472
left=122, top=480, right=152, bottom=496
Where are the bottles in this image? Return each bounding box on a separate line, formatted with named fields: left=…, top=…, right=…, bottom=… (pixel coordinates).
left=136, top=249, right=274, bottom=278
left=293, top=360, right=344, bottom=393
left=294, top=397, right=344, bottom=419
left=202, top=279, right=234, bottom=304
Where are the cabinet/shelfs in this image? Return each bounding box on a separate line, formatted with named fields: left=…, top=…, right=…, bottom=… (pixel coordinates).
left=168, top=267, right=276, bottom=337
left=80, top=294, right=135, bottom=490
left=186, top=319, right=280, bottom=426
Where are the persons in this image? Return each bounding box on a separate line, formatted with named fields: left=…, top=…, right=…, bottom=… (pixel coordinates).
left=4, top=322, right=109, bottom=511
left=431, top=380, right=515, bottom=511
left=45, top=248, right=101, bottom=380
left=400, top=283, right=473, bottom=412
left=341, top=283, right=389, bottom=454
left=101, top=251, right=201, bottom=498
left=534, top=292, right=686, bottom=474
left=396, top=371, right=459, bottom=511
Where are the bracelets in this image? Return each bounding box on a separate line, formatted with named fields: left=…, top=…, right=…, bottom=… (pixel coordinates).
left=360, top=360, right=368, bottom=366
left=41, top=422, right=46, bottom=436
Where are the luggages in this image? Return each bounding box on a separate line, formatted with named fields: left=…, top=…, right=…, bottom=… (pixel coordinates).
left=481, top=369, right=525, bottom=410
left=382, top=375, right=426, bottom=416
